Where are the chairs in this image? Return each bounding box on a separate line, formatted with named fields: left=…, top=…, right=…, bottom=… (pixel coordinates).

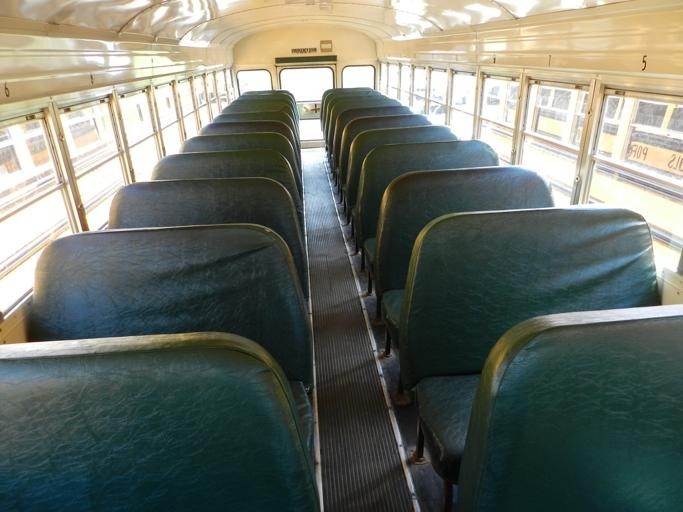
left=3, top=90, right=319, bottom=508
left=319, top=89, right=682, bottom=507
